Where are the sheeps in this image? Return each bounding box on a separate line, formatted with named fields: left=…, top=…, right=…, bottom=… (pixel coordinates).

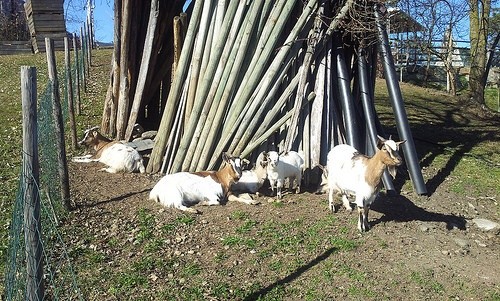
left=325, top=133, right=407, bottom=232
left=148, top=152, right=261, bottom=213
left=232, top=150, right=270, bottom=199
left=70, top=126, right=146, bottom=174
left=262, top=149, right=303, bottom=200
